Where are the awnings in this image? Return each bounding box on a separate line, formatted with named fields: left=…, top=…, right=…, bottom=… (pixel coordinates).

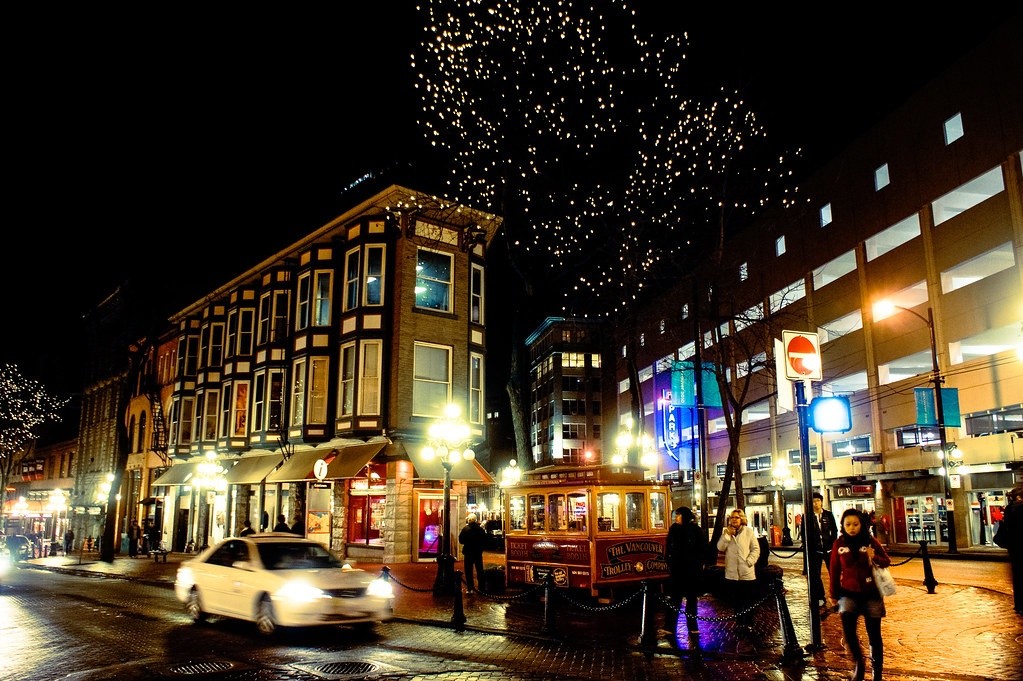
left=151, top=463, right=202, bottom=486
left=224, top=453, right=288, bottom=484
left=266, top=448, right=335, bottom=483
left=706, top=433, right=1023, bottom=492
left=324, top=443, right=387, bottom=478
left=448, top=454, right=484, bottom=482
left=402, top=441, right=447, bottom=480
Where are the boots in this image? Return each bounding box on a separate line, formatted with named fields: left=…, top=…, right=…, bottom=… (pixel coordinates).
left=873, top=661, right=882, bottom=681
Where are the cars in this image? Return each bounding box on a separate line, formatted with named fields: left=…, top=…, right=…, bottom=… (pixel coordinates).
left=0, top=534, right=32, bottom=559
left=175, top=532, right=396, bottom=638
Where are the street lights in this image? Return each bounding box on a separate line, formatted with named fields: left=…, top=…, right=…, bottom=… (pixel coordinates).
left=467, top=459, right=524, bottom=528
left=610, top=417, right=661, bottom=528
left=43, top=488, right=66, bottom=556
left=876, top=299, right=957, bottom=553
left=420, top=402, right=475, bottom=595
left=11, top=497, right=30, bottom=535
left=192, top=450, right=228, bottom=552
left=95, top=473, right=121, bottom=555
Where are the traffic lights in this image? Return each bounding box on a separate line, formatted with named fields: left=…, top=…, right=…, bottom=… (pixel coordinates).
left=810, top=396, right=853, bottom=431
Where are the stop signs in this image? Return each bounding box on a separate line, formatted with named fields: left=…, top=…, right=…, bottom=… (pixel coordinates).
left=782, top=329, right=823, bottom=381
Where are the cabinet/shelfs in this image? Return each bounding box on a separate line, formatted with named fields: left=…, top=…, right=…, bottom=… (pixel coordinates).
left=905, top=498, right=948, bottom=543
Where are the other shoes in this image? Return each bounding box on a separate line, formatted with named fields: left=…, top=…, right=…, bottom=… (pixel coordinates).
left=477, top=588, right=488, bottom=593
left=657, top=630, right=672, bottom=635
left=687, top=630, right=700, bottom=634
left=132, top=556, right=135, bottom=559
left=852, top=663, right=865, bottom=681
left=465, top=590, right=473, bottom=593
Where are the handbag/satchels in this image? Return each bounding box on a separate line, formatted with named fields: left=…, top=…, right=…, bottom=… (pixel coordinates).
left=867, top=535, right=895, bottom=596
left=993, top=520, right=1009, bottom=549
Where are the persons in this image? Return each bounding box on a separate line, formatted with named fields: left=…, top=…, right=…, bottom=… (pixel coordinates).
left=291, top=515, right=305, bottom=536
left=458, top=513, right=488, bottom=594
left=127, top=519, right=160, bottom=559
left=480, top=516, right=514, bottom=536
left=65, top=530, right=74, bottom=555
left=992, top=488, right=1023, bottom=611
left=830, top=509, right=886, bottom=681
left=801, top=493, right=838, bottom=608
left=659, top=508, right=710, bottom=633
left=5, top=530, right=43, bottom=548
left=87, top=535, right=103, bottom=552
left=273, top=515, right=290, bottom=534
left=240, top=520, right=256, bottom=536
left=717, top=509, right=760, bottom=633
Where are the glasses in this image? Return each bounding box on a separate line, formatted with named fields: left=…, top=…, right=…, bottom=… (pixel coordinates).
left=729, top=516, right=740, bottom=520
